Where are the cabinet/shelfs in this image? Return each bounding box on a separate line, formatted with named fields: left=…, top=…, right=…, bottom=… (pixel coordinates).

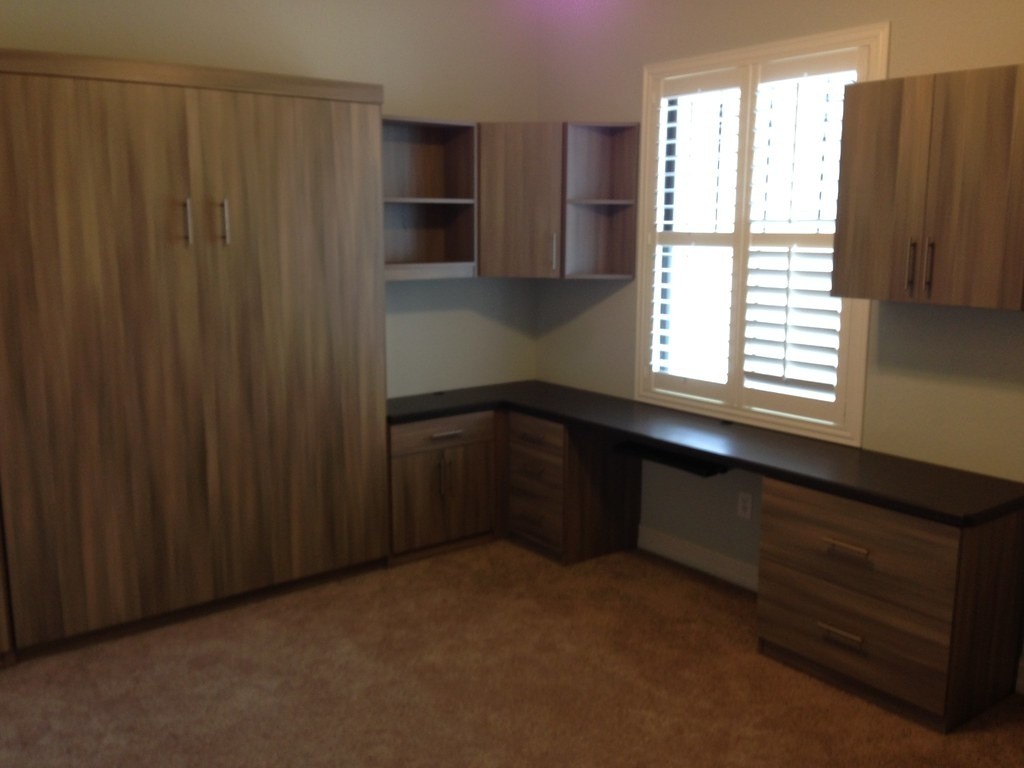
left=390, top=405, right=642, bottom=562
left=1, top=44, right=389, bottom=667
left=383, top=115, right=640, bottom=281
left=753, top=474, right=1024, bottom=731
left=830, top=63, right=1023, bottom=313
